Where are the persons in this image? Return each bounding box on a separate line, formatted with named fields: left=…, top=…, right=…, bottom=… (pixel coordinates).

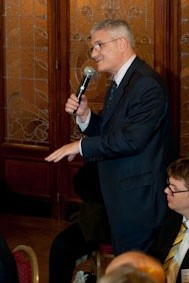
left=45, top=18, right=172, bottom=283
left=145, top=156, right=189, bottom=283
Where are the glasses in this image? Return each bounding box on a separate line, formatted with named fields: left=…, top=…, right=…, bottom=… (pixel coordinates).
left=167, top=178, right=189, bottom=197
left=91, top=38, right=122, bottom=53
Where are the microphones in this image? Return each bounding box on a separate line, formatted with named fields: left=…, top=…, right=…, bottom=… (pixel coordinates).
left=73, top=66, right=94, bottom=114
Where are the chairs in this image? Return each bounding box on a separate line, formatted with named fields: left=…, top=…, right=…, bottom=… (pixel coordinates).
left=11, top=245, right=39, bottom=283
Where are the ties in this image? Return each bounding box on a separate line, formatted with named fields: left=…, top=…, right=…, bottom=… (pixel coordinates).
left=161, top=222, right=188, bottom=271
left=108, top=80, right=117, bottom=110
